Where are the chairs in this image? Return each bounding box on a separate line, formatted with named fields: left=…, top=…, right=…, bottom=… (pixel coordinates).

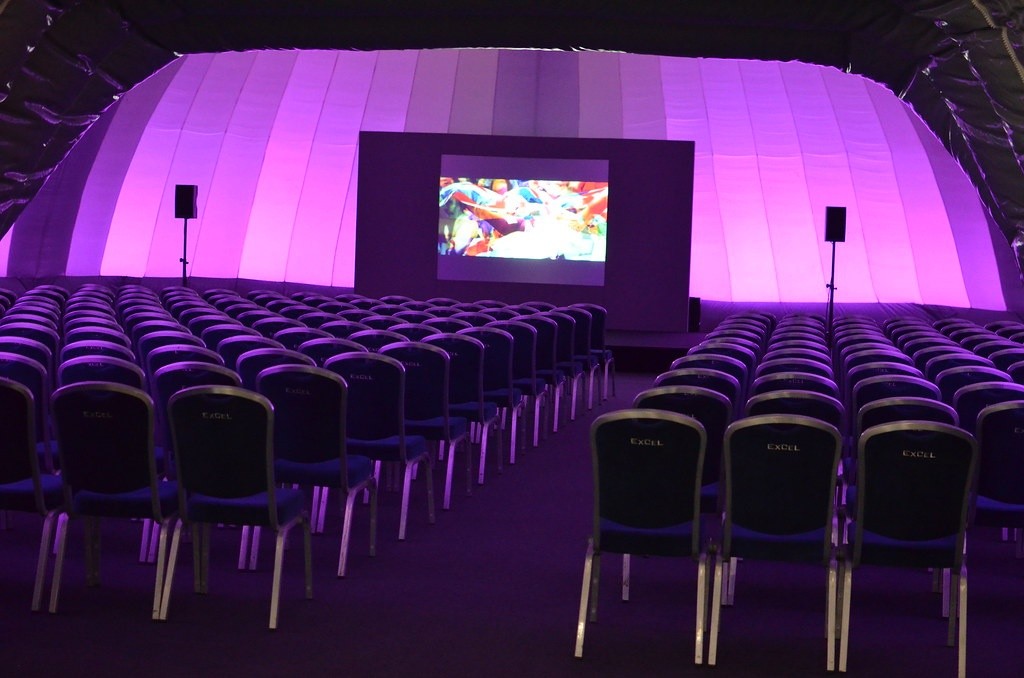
left=0, top=283, right=1024, bottom=678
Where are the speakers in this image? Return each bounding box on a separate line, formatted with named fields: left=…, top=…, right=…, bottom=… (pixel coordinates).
left=175, top=185, right=198, bottom=219
left=688, top=297, right=701, bottom=332
left=825, top=206, right=846, bottom=242
